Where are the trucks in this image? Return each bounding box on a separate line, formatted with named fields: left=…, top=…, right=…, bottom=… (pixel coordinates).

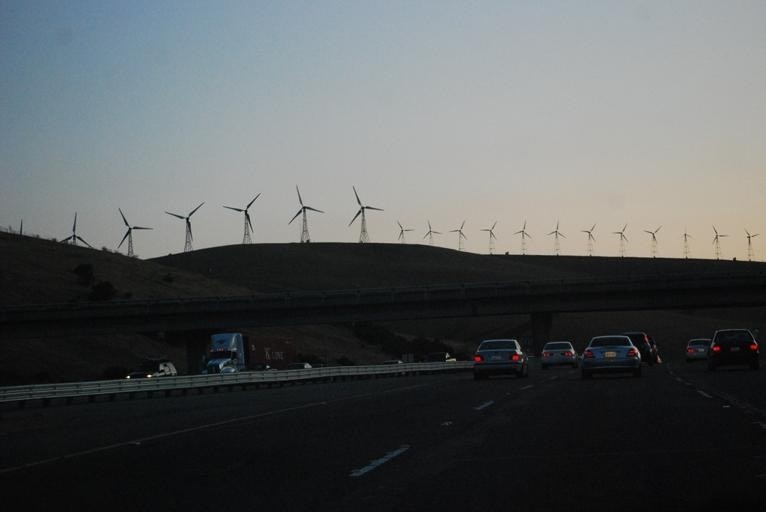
left=205, top=333, right=297, bottom=373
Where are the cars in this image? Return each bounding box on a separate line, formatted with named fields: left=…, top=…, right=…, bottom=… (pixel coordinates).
left=125, top=362, right=177, bottom=379
left=287, top=352, right=457, bottom=378
left=685, top=338, right=712, bottom=363
left=473, top=338, right=530, bottom=379
left=541, top=341, right=580, bottom=370
left=706, top=328, right=761, bottom=372
left=582, top=331, right=661, bottom=380
